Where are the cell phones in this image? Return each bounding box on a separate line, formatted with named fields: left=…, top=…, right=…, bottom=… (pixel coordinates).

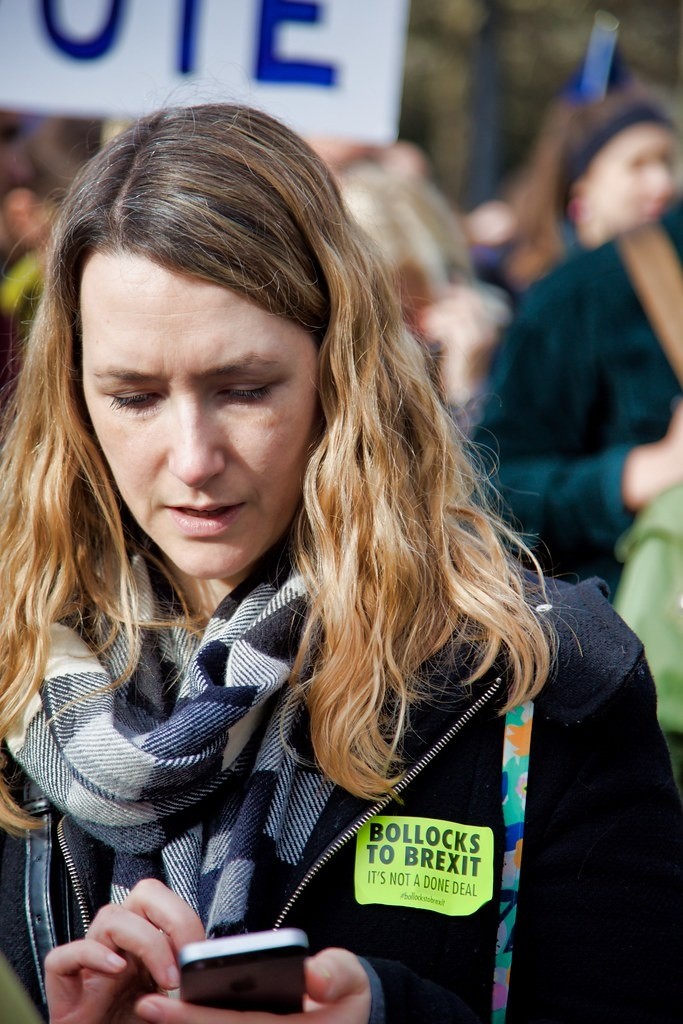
left=178, top=927, right=308, bottom=1017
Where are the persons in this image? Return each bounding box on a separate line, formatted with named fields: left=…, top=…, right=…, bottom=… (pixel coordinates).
left=311, top=139, right=503, bottom=407
left=0, top=114, right=99, bottom=405
left=0, top=104, right=683, bottom=1024
left=477, top=206, right=683, bottom=608
left=463, top=48, right=679, bottom=304
